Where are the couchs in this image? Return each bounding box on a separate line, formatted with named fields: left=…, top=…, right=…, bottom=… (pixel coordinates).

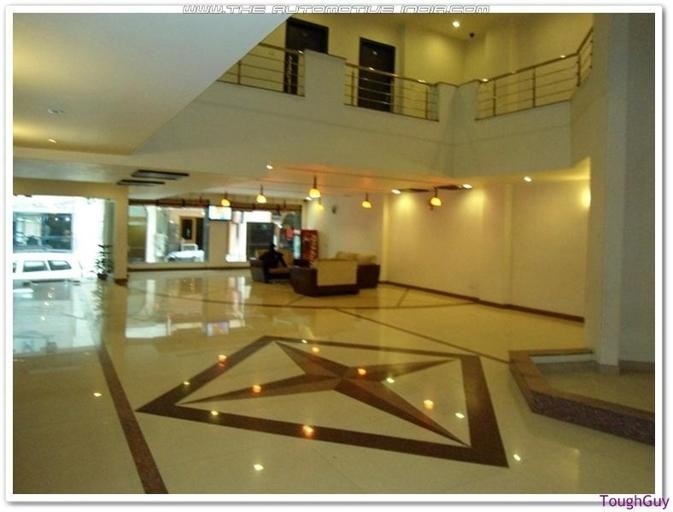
left=249, top=248, right=380, bottom=297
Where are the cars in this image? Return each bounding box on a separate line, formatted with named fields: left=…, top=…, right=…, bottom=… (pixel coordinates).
left=161, top=250, right=206, bottom=263
left=82, top=258, right=102, bottom=277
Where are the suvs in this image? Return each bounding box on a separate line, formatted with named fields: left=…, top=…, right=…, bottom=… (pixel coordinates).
left=13, top=249, right=81, bottom=289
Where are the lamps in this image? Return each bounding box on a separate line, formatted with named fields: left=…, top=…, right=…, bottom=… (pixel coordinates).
left=428, top=187, right=442, bottom=207
left=221, top=193, right=230, bottom=206
left=256, top=185, right=267, bottom=203
left=362, top=192, right=373, bottom=209
left=309, top=175, right=320, bottom=198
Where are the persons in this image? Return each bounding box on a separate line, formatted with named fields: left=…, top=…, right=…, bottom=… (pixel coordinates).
left=258, top=243, right=287, bottom=281
